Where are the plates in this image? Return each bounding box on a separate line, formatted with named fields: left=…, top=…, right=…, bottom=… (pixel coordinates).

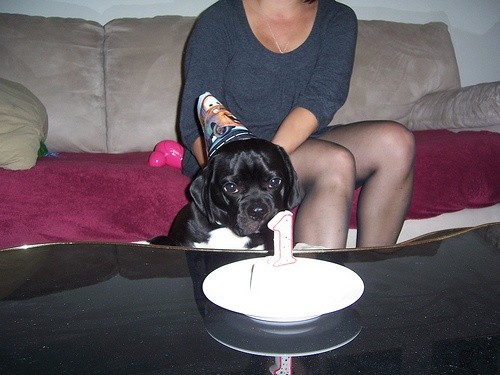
left=203, top=257, right=366, bottom=324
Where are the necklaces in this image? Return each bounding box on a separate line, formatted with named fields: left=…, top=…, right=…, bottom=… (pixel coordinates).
left=267, top=19, right=290, bottom=54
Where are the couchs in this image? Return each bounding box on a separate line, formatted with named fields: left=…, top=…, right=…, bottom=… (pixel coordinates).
left=0, top=12, right=500, bottom=249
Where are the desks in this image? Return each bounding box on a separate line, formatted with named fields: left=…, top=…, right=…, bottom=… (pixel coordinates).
left=0, top=221, right=500, bottom=375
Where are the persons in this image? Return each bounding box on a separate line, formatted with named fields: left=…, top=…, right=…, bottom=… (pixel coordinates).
left=179, top=0, right=415, bottom=250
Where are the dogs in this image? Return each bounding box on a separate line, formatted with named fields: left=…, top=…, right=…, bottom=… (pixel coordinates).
left=131, top=138, right=301, bottom=249
